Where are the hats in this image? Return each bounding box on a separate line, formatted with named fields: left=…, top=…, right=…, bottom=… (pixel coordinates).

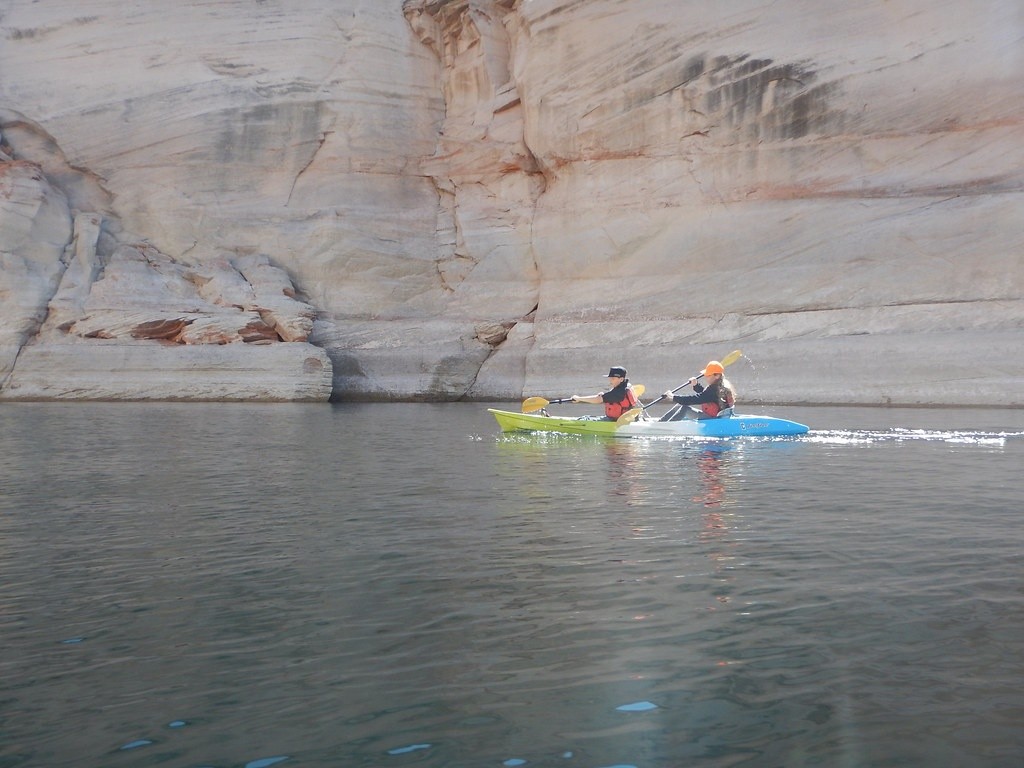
left=700, top=361, right=724, bottom=376
left=602, top=366, right=626, bottom=378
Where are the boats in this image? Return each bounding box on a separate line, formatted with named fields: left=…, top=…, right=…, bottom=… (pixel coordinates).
left=486, top=406, right=811, bottom=439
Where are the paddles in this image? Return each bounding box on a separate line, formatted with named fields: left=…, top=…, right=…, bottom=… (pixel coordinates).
left=615, top=351, right=743, bottom=429
left=521, top=383, right=646, bottom=413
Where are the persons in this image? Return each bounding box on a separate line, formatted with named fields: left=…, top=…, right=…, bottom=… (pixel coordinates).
left=542, top=366, right=640, bottom=424
left=640, top=360, right=736, bottom=422
